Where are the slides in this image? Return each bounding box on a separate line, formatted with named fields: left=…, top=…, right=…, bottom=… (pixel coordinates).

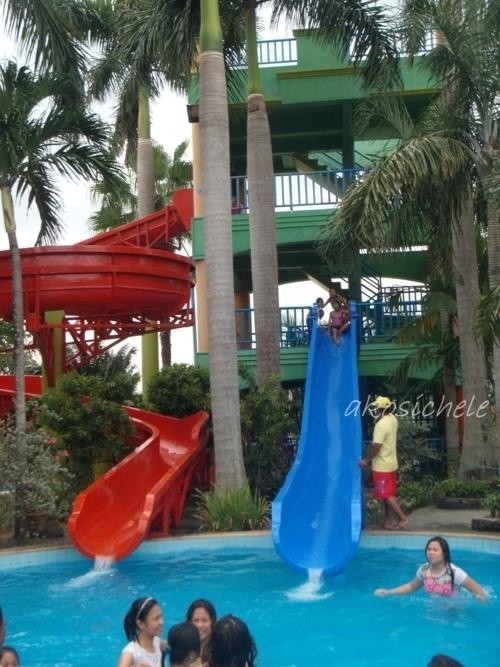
left=2, top=189, right=210, bottom=560
left=273, top=299, right=363, bottom=575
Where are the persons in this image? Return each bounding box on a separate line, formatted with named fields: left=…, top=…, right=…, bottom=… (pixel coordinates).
left=118, top=596, right=170, bottom=667
left=306, top=287, right=350, bottom=346
left=161, top=623, right=211, bottom=667
left=0, top=647, right=20, bottom=667
left=374, top=537, right=487, bottom=599
left=427, top=654, right=465, bottom=667
left=232, top=192, right=245, bottom=214
left=0, top=608, right=6, bottom=646
left=359, top=396, right=411, bottom=530
left=201, top=614, right=258, bottom=667
left=186, top=599, right=216, bottom=657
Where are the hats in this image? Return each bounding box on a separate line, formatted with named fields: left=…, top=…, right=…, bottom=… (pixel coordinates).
left=371, top=396, right=391, bottom=408
